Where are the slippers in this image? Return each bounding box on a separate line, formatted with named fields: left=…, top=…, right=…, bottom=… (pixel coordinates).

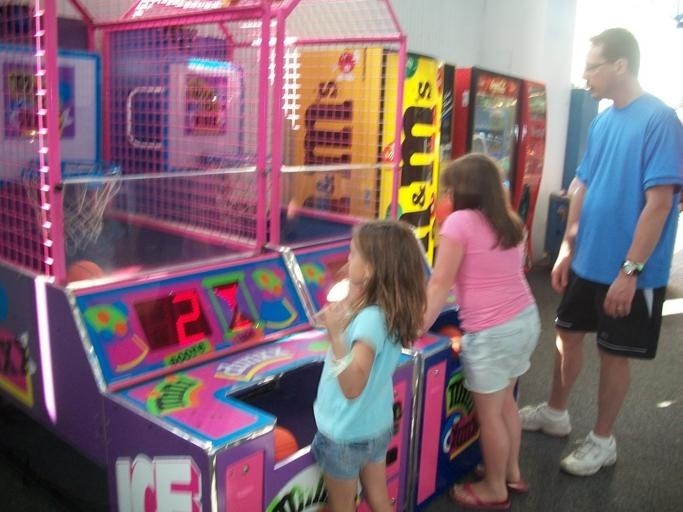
left=477, top=466, right=529, bottom=494
left=449, top=484, right=512, bottom=510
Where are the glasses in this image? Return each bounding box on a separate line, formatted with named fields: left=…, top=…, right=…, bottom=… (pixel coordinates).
left=585, top=59, right=611, bottom=73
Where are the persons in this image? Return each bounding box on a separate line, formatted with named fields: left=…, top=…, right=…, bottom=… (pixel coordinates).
left=519, top=28, right=683, bottom=482
left=310, top=219, right=428, bottom=512
left=416, top=152, right=541, bottom=510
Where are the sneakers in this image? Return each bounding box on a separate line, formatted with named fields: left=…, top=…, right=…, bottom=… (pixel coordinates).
left=558, top=431, right=618, bottom=476
left=516, top=402, right=573, bottom=438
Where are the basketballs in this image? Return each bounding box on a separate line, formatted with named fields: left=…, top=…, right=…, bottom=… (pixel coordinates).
left=65, top=259, right=104, bottom=286
left=437, top=326, right=462, bottom=361
left=271, top=428, right=298, bottom=466
left=433, top=194, right=453, bottom=231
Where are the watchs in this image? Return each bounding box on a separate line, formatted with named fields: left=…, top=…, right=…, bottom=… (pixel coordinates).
left=621, top=260, right=645, bottom=277
